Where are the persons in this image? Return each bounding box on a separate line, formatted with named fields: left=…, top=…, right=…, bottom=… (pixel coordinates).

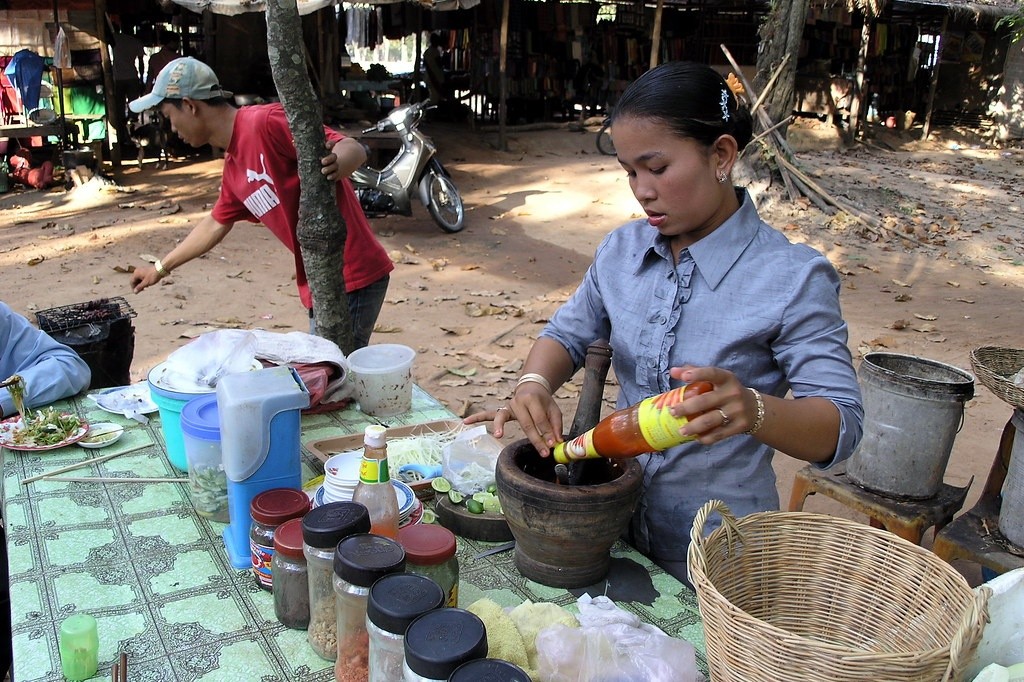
left=463, top=61, right=866, bottom=590
left=0, top=302, right=90, bottom=418
left=112, top=16, right=146, bottom=145
left=423, top=33, right=444, bottom=102
left=128, top=56, right=395, bottom=349
left=146, top=31, right=182, bottom=94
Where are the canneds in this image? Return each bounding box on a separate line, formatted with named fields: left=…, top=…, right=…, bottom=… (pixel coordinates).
left=397, top=524, right=460, bottom=610
left=249, top=488, right=311, bottom=630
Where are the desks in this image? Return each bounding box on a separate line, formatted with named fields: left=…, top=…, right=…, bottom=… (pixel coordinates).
left=784, top=461, right=971, bottom=549
left=0, top=363, right=708, bottom=682
left=932, top=510, right=1024, bottom=578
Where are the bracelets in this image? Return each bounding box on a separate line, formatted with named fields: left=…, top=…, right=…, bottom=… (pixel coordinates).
left=155, top=260, right=171, bottom=276
left=515, top=373, right=551, bottom=392
left=746, top=388, right=765, bottom=435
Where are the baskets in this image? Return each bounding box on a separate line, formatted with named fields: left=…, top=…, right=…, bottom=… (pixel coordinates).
left=685, top=500, right=993, bottom=682
left=969, top=345, right=1024, bottom=410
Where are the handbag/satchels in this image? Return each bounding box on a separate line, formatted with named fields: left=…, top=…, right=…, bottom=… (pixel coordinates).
left=163, top=329, right=352, bottom=412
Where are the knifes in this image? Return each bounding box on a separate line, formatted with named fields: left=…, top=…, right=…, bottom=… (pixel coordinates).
left=476, top=540, right=515, bottom=559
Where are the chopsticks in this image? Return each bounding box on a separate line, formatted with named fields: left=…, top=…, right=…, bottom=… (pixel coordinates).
left=0, top=379, right=20, bottom=390
left=21, top=442, right=189, bottom=485
left=111, top=652, right=127, bottom=682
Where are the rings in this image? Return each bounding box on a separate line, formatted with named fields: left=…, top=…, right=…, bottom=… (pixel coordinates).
left=497, top=407, right=508, bottom=411
left=719, top=410, right=729, bottom=425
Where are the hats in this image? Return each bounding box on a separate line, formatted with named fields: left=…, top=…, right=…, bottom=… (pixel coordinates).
left=129, top=56, right=234, bottom=113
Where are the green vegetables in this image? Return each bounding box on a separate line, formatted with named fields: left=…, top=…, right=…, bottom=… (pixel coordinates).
left=7, top=375, right=83, bottom=445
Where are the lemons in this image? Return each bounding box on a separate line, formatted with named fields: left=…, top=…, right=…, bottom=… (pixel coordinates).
left=424, top=477, right=501, bottom=524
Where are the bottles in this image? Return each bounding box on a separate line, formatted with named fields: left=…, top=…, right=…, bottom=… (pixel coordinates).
left=249, top=489, right=310, bottom=590
left=272, top=517, right=308, bottom=630
left=448, top=659, right=532, bottom=682
left=398, top=524, right=460, bottom=608
left=350, top=426, right=400, bottom=543
left=403, top=607, right=488, bottom=682
left=301, top=501, right=371, bottom=662
left=334, top=534, right=405, bottom=682
left=554, top=379, right=714, bottom=464
left=365, top=573, right=445, bottom=682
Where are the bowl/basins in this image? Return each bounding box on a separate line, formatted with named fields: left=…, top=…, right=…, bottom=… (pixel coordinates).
left=346, top=344, right=416, bottom=417
left=180, top=394, right=230, bottom=523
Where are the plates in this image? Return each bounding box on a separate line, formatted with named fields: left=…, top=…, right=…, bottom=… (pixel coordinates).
left=96, top=386, right=158, bottom=415
left=311, top=453, right=425, bottom=529
left=1, top=414, right=124, bottom=451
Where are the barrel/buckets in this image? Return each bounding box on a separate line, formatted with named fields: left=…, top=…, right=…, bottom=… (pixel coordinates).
left=998, top=409, right=1024, bottom=549
left=846, top=352, right=976, bottom=497
left=147, top=356, right=262, bottom=473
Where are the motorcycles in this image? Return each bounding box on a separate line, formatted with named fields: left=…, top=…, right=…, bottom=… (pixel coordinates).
left=344, top=94, right=465, bottom=234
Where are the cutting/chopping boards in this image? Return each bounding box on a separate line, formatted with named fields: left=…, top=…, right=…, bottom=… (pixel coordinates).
left=435, top=492, right=512, bottom=541
